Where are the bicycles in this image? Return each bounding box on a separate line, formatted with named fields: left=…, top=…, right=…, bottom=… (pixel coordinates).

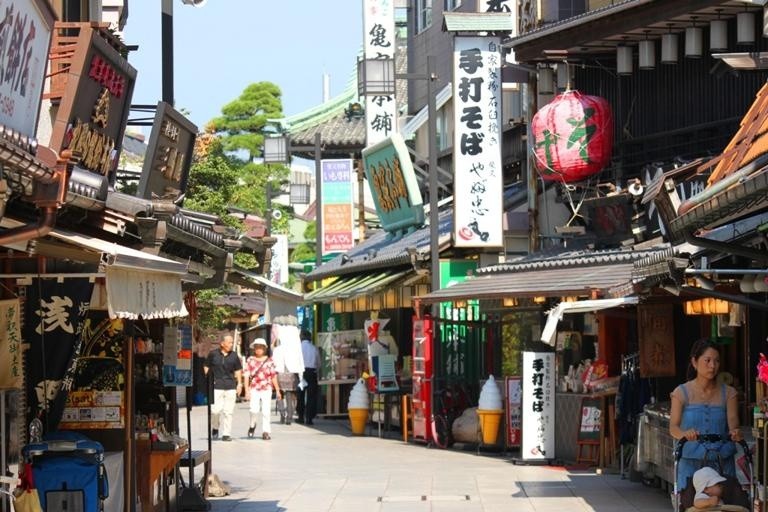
left=430, top=376, right=462, bottom=449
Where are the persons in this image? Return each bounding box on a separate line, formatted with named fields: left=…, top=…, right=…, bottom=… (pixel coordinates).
left=270, top=338, right=300, bottom=425
left=669, top=340, right=743, bottom=499
left=203, top=333, right=244, bottom=442
left=692, top=466, right=728, bottom=512
left=243, top=338, right=282, bottom=440
left=294, top=329, right=323, bottom=425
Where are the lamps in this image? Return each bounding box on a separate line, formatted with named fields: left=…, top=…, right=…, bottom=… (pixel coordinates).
left=751, top=272, right=767, bottom=294
left=658, top=277, right=680, bottom=297
left=536, top=58, right=555, bottom=97
left=739, top=272, right=756, bottom=294
left=691, top=273, right=715, bottom=291
left=616, top=3, right=757, bottom=77
left=554, top=60, right=575, bottom=93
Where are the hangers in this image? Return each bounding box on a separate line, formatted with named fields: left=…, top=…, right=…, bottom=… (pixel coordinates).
left=624, top=350, right=640, bottom=373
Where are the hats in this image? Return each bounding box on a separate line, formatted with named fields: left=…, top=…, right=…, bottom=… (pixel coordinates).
left=693, top=467, right=726, bottom=495
left=249, top=338, right=268, bottom=349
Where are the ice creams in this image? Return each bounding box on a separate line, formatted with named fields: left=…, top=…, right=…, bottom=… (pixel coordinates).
left=476, top=372, right=505, bottom=443
left=346, top=379, right=371, bottom=435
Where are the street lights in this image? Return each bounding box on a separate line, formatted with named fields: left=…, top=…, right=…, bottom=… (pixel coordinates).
left=353, top=45, right=444, bottom=421
left=259, top=127, right=323, bottom=290
left=259, top=176, right=312, bottom=241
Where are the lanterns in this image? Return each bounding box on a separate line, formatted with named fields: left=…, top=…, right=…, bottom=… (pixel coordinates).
left=531, top=59, right=614, bottom=185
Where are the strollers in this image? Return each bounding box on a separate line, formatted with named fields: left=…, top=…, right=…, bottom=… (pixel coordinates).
left=20, top=432, right=108, bottom=512
left=670, top=429, right=761, bottom=512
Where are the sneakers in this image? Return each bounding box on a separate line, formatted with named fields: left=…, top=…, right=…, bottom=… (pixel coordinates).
left=249, top=427, right=255, bottom=437
left=212, top=429, right=231, bottom=441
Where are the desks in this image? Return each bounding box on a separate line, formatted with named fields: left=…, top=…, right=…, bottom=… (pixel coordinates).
left=367, top=387, right=413, bottom=439
left=135, top=434, right=190, bottom=512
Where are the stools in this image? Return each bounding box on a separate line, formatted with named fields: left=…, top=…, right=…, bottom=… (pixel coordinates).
left=174, top=450, right=211, bottom=506
